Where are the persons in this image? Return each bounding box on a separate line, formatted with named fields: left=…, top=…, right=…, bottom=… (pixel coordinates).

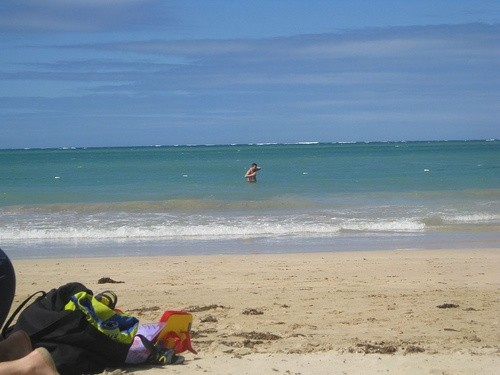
left=0, top=250, right=60, bottom=375
left=245, top=162, right=262, bottom=182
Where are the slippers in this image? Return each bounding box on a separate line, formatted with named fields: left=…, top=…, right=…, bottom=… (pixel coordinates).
left=146, top=349, right=185, bottom=365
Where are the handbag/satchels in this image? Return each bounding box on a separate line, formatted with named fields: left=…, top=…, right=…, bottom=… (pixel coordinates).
left=0, top=281, right=139, bottom=374
